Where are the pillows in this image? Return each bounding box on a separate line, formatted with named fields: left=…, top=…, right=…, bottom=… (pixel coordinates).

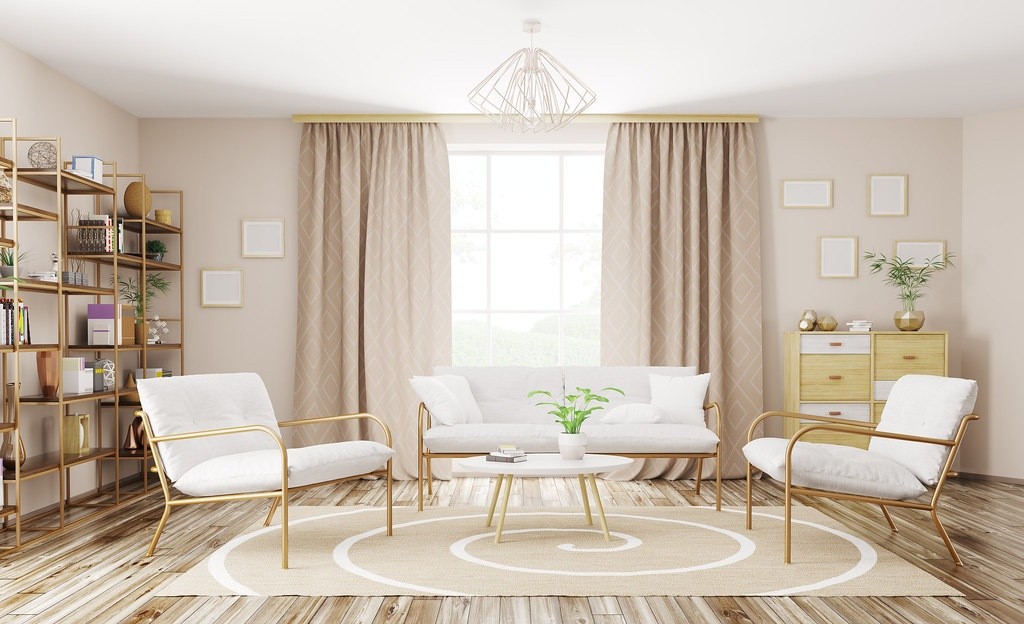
left=648, top=371, right=712, bottom=428
left=408, top=375, right=485, bottom=428
left=598, top=400, right=661, bottom=424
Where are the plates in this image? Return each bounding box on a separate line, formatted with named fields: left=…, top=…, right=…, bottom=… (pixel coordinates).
left=124, top=252, right=158, bottom=258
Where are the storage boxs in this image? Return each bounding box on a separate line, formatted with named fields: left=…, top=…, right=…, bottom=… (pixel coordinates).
left=70, top=156, right=103, bottom=183
left=86, top=304, right=123, bottom=346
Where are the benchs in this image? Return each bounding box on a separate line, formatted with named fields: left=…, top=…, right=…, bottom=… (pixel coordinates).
left=408, top=364, right=721, bottom=511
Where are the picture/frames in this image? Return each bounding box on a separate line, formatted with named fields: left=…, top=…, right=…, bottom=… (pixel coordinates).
left=781, top=177, right=832, bottom=210
left=896, top=242, right=949, bottom=271
left=819, top=234, right=858, bottom=280
left=867, top=174, right=908, bottom=218
left=239, top=218, right=286, bottom=260
left=199, top=269, right=244, bottom=308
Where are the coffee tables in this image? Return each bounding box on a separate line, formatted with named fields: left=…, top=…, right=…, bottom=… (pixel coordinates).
left=457, top=450, right=633, bottom=545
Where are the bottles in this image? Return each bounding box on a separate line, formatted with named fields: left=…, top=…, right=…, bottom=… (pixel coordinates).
left=1, top=383, right=26, bottom=470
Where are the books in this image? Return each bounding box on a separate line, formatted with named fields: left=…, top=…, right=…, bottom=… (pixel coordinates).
left=28, top=271, right=58, bottom=282
left=846, top=320, right=873, bottom=331
left=135, top=367, right=171, bottom=378
left=88, top=303, right=137, bottom=345
left=77, top=214, right=124, bottom=253
left=0, top=298, right=32, bottom=345
left=63, top=356, right=103, bottom=396
left=64, top=169, right=92, bottom=180
left=486, top=445, right=528, bottom=463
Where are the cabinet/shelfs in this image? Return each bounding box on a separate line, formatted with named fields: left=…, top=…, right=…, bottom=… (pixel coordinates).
left=785, top=329, right=949, bottom=447
left=0, top=118, right=184, bottom=560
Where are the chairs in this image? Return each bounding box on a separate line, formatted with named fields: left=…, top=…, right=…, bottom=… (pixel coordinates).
left=135, top=371, right=397, bottom=569
left=742, top=375, right=980, bottom=568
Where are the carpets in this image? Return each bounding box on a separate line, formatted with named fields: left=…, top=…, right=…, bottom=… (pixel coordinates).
left=153, top=506, right=967, bottom=597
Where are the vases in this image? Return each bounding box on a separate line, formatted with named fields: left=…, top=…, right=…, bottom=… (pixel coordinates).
left=1, top=382, right=26, bottom=471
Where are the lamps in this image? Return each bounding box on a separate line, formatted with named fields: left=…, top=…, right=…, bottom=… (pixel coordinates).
left=465, top=20, right=595, bottom=135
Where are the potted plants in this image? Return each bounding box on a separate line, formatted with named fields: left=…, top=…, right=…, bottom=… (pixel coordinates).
left=110, top=271, right=173, bottom=346
left=143, top=236, right=168, bottom=263
left=0, top=243, right=31, bottom=278
left=526, top=381, right=626, bottom=462
left=862, top=248, right=956, bottom=333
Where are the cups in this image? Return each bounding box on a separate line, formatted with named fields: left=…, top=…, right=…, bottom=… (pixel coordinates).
left=154, top=210, right=172, bottom=227
